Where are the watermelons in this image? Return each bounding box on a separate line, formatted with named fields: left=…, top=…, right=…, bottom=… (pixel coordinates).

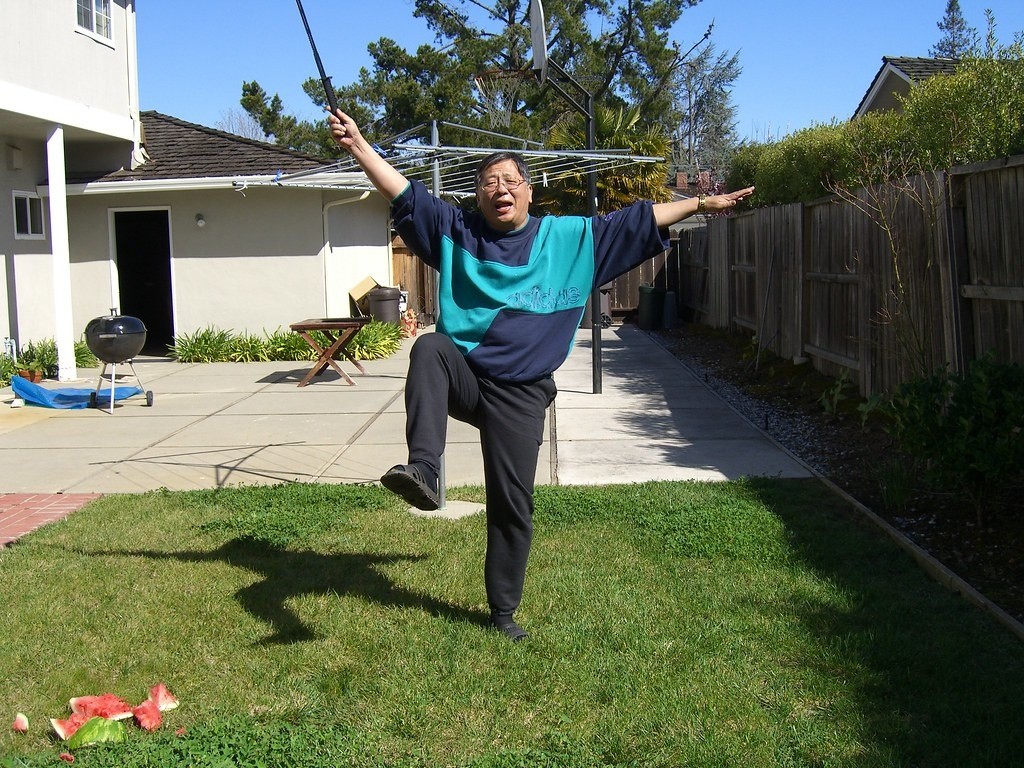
left=14, top=682, right=180, bottom=749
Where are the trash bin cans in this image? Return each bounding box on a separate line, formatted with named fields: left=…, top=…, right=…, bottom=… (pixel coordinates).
left=638, top=286, right=666, bottom=330
left=366, top=287, right=401, bottom=329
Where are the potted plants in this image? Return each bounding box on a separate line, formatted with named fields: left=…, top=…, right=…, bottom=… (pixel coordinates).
left=13, top=360, right=42, bottom=384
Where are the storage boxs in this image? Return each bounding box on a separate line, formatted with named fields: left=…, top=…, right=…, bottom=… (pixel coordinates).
left=349, top=276, right=382, bottom=317
left=401, top=318, right=417, bottom=339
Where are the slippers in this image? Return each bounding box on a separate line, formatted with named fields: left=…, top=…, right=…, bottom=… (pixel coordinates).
left=490, top=614, right=529, bottom=644
left=380, top=464, right=439, bottom=511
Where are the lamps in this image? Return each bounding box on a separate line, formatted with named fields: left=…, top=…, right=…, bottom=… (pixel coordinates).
left=196, top=214, right=206, bottom=226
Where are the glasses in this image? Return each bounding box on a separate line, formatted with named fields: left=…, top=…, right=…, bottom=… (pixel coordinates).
left=478, top=178, right=527, bottom=193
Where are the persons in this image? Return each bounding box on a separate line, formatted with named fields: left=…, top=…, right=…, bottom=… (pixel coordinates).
left=323, top=103, right=754, bottom=639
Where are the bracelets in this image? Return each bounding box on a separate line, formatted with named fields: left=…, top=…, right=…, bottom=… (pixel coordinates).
left=697, top=193, right=705, bottom=212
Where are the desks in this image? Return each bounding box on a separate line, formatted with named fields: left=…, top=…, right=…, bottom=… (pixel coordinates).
left=290, top=317, right=373, bottom=387
